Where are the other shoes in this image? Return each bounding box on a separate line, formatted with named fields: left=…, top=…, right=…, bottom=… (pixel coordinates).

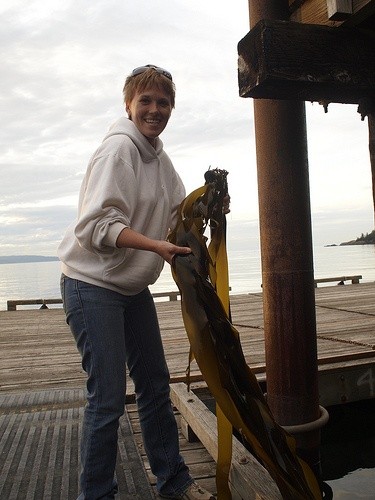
left=171, top=476, right=216, bottom=499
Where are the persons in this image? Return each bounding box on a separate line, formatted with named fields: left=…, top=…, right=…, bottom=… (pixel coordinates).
left=55, top=65, right=218, bottom=500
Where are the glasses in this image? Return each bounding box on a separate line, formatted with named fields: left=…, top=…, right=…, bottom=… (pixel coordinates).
left=132, top=66, right=173, bottom=80
